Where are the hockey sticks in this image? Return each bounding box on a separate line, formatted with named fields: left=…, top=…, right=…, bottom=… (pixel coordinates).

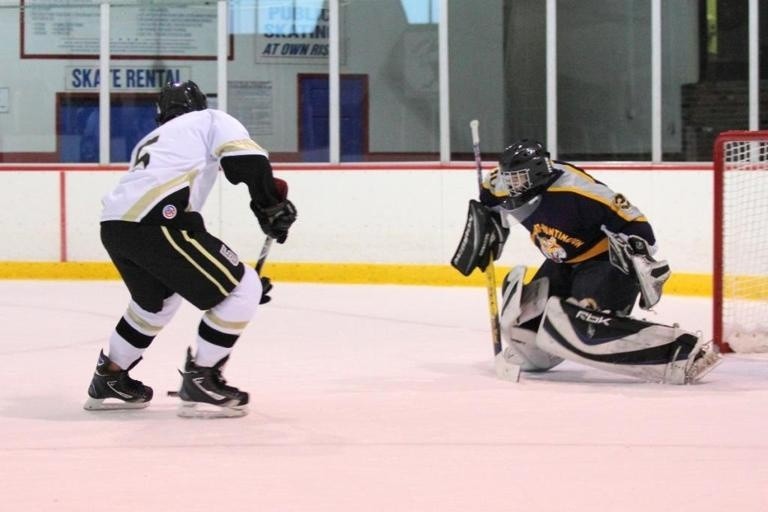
left=469, top=119, right=521, bottom=385
left=167, top=229, right=273, bottom=399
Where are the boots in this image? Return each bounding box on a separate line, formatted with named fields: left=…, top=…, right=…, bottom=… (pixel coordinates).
left=176, top=346, right=249, bottom=407
left=86, top=348, right=154, bottom=404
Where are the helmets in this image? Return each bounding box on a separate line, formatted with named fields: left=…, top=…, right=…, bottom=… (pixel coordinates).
left=156, top=78, right=207, bottom=121
left=499, top=138, right=556, bottom=188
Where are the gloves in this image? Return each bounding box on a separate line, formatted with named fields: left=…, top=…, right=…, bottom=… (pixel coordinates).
left=248, top=200, right=296, bottom=246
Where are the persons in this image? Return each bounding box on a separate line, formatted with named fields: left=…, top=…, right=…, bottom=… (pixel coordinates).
left=452, top=140, right=722, bottom=385
left=87, top=82, right=296, bottom=406
